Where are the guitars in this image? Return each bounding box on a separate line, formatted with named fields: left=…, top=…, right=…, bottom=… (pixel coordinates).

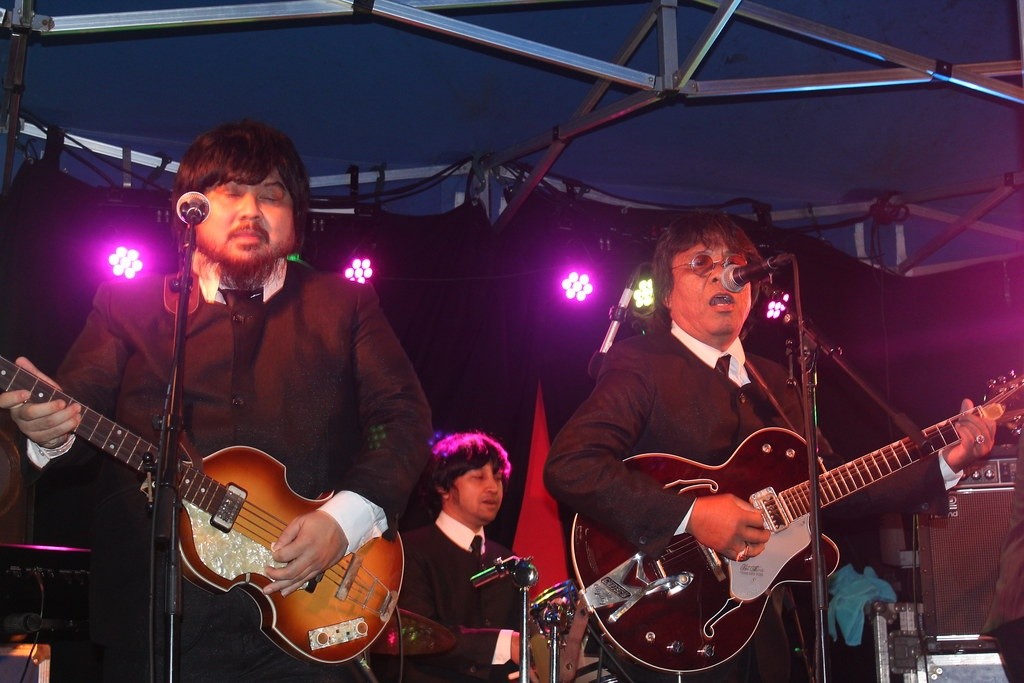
left=568, top=370, right=1024, bottom=676
left=0, top=355, right=407, bottom=667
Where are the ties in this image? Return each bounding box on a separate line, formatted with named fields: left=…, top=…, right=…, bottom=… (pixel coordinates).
left=714, top=354, right=732, bottom=377
left=218, top=285, right=264, bottom=315
left=470, top=535, right=483, bottom=557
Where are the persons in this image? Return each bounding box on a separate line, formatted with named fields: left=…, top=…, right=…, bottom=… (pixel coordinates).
left=0, top=122, right=432, bottom=683
left=366, top=430, right=540, bottom=683
left=543, top=209, right=997, bottom=683
left=980, top=422, right=1024, bottom=683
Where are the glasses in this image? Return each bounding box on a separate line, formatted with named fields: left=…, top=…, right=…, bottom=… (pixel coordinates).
left=671, top=253, right=748, bottom=278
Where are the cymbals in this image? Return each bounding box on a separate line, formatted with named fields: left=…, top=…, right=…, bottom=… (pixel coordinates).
left=369, top=606, right=458, bottom=657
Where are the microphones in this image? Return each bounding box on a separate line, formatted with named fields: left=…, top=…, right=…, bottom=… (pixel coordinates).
left=588, top=265, right=641, bottom=380
left=720, top=253, right=794, bottom=294
left=176, top=192, right=211, bottom=225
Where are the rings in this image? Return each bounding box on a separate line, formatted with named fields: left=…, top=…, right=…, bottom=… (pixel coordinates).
left=736, top=545, right=749, bottom=562
left=975, top=435, right=985, bottom=445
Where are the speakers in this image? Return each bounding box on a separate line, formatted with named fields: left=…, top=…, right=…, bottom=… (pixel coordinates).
left=913, top=444, right=1021, bottom=653
